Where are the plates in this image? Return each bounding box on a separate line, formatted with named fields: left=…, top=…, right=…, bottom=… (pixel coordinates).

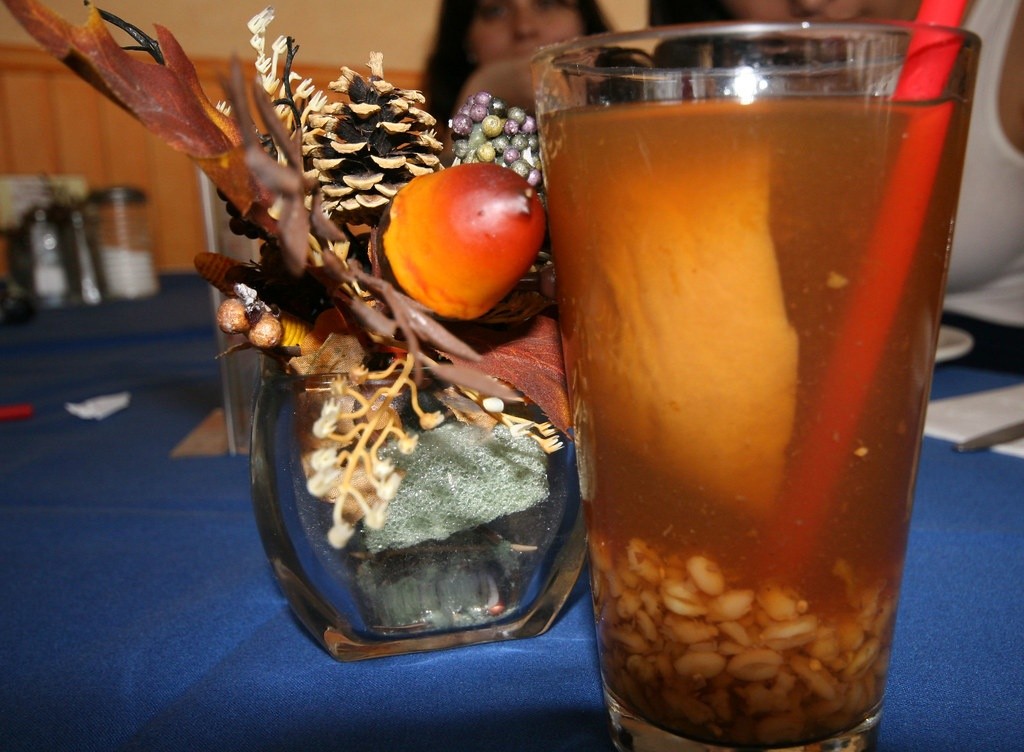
left=934, top=324, right=974, bottom=362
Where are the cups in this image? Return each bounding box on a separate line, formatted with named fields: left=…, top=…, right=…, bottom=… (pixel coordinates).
left=87, top=184, right=160, bottom=300
left=519, top=14, right=984, bottom=752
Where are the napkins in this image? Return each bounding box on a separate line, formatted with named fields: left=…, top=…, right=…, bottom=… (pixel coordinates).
left=922, top=386, right=1024, bottom=460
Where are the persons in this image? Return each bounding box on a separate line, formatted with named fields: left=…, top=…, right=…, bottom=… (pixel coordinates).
left=648, top=0, right=1024, bottom=380
left=415, top=0, right=644, bottom=166
